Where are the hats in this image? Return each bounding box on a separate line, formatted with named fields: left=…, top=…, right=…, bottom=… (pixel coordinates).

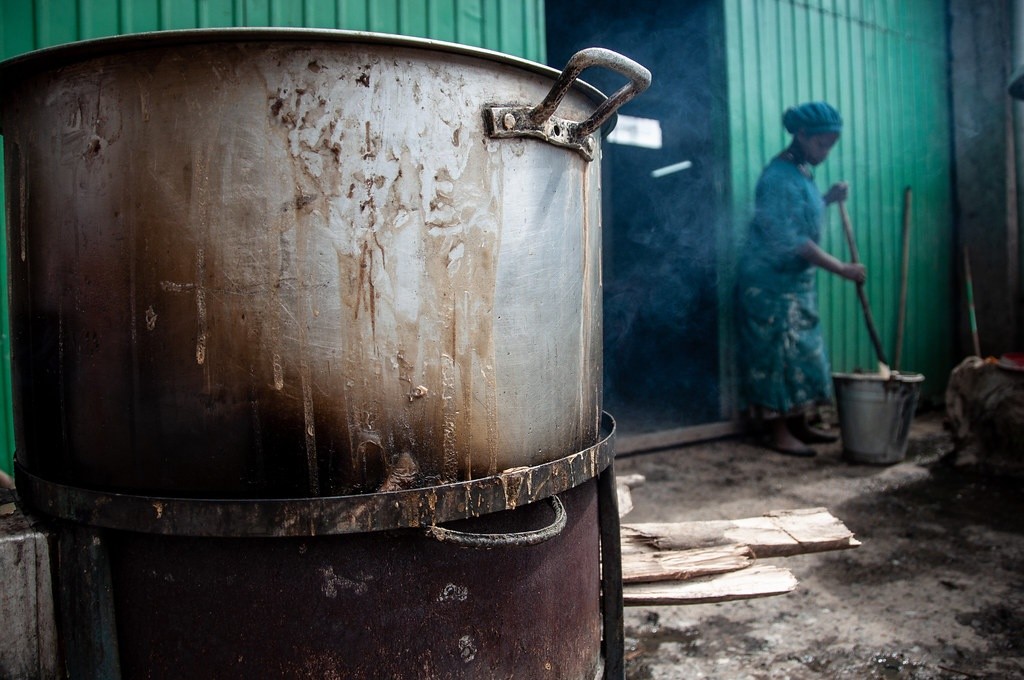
left=783, top=102, right=843, bottom=134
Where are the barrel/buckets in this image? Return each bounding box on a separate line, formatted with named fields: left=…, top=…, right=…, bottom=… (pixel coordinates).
left=832, top=370, right=925, bottom=465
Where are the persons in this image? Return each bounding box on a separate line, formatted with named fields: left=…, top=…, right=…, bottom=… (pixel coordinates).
left=736, top=102, right=868, bottom=457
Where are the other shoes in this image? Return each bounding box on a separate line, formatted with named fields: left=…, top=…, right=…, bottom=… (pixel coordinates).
left=764, top=443, right=817, bottom=458
left=798, top=431, right=839, bottom=445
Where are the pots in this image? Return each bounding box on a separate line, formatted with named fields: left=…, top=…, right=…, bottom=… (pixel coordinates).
left=0, top=22, right=654, bottom=539
